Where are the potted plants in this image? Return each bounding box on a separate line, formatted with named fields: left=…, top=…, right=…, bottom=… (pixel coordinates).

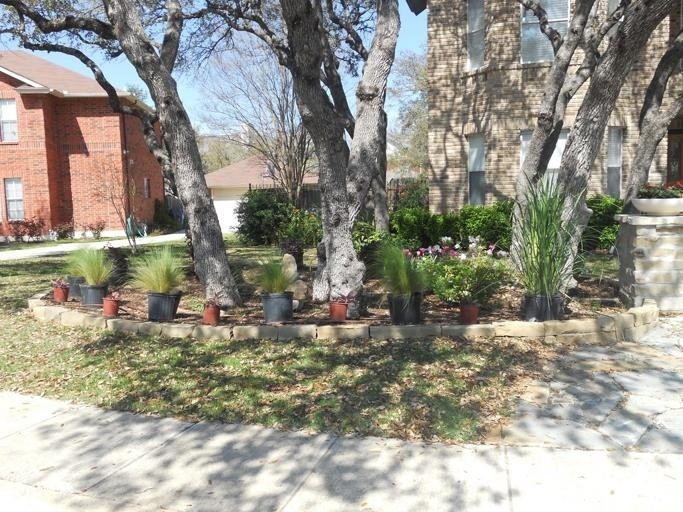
left=436, top=264, right=499, bottom=323
left=278, top=211, right=315, bottom=270
left=500, top=170, right=586, bottom=321
left=248, top=246, right=300, bottom=324
left=370, top=236, right=432, bottom=324
left=58, top=253, right=86, bottom=297
left=70, top=244, right=120, bottom=306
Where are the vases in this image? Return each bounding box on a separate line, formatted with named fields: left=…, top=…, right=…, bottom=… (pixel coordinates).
left=128, top=241, right=196, bottom=323
left=202, top=301, right=221, bottom=324
left=329, top=302, right=348, bottom=322
left=55, top=279, right=69, bottom=302
left=101, top=291, right=120, bottom=318
left=630, top=198, right=682, bottom=215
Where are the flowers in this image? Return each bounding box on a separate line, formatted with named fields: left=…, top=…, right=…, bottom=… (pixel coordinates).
left=639, top=180, right=682, bottom=197
